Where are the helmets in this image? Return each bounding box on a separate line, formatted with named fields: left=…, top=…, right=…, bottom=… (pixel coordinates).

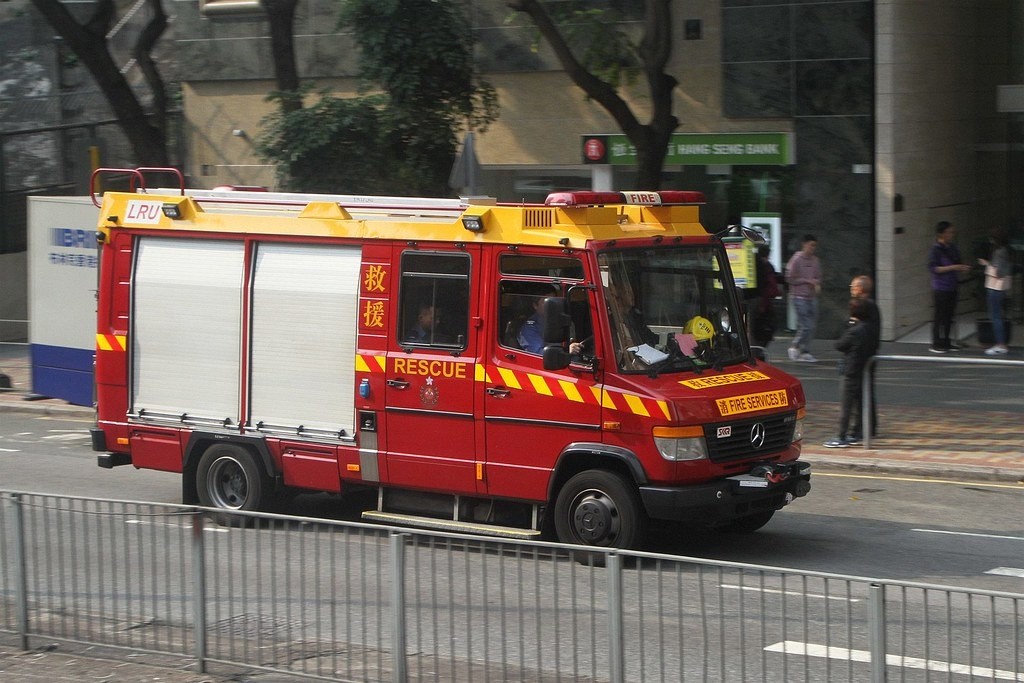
left=682, top=316, right=714, bottom=342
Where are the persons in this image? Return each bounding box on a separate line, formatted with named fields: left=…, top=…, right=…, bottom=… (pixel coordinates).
left=925, top=218, right=971, bottom=357
left=743, top=243, right=783, bottom=356
left=406, top=297, right=455, bottom=346
left=838, top=275, right=883, bottom=438
left=516, top=284, right=585, bottom=359
left=979, top=226, right=1013, bottom=356
left=823, top=294, right=877, bottom=450
left=784, top=237, right=828, bottom=364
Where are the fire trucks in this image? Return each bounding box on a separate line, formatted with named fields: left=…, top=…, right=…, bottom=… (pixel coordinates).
left=81, top=163, right=818, bottom=568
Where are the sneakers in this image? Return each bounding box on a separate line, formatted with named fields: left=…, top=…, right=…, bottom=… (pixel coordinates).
left=823, top=433, right=858, bottom=448
left=983, top=344, right=1008, bottom=356
left=928, top=343, right=960, bottom=353
left=787, top=347, right=818, bottom=362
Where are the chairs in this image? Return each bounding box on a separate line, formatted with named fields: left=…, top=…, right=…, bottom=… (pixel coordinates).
left=505, top=313, right=529, bottom=349
